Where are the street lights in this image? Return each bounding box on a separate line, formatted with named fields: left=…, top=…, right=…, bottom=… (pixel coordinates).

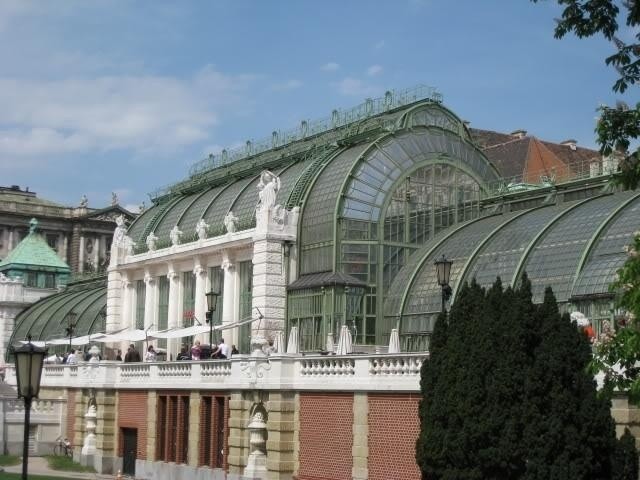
left=282, top=240, right=293, bottom=352
left=206, top=291, right=219, bottom=354
left=434, top=258, right=453, bottom=314
left=11, top=336, right=49, bottom=480
left=66, top=312, right=76, bottom=350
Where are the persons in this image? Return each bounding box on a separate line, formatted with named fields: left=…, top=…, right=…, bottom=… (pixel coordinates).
left=116, top=349, right=123, bottom=361
left=210, top=338, right=228, bottom=359
left=191, top=340, right=202, bottom=360
left=125, top=344, right=142, bottom=362
left=177, top=347, right=191, bottom=359
left=231, top=345, right=239, bottom=354
left=145, top=345, right=158, bottom=362
left=54, top=343, right=109, bottom=364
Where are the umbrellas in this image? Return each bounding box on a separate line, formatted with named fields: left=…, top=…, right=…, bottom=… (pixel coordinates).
left=43, top=354, right=64, bottom=362
left=327, top=324, right=353, bottom=356
left=387, top=329, right=401, bottom=354
left=273, top=327, right=300, bottom=354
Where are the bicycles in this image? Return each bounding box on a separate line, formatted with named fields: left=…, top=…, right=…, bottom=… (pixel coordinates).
left=54, top=436, right=73, bottom=459
